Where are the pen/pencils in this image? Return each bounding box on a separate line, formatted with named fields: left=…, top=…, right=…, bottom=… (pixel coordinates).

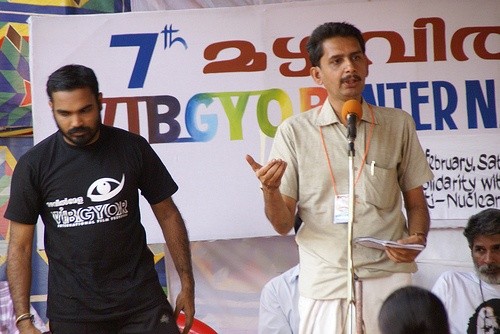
left=370, top=160, right=377, bottom=177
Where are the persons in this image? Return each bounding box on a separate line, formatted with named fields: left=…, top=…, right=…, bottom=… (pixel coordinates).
left=378, top=285, right=451, bottom=334
left=258, top=211, right=303, bottom=334
left=432, top=208, right=500, bottom=334
left=3, top=64, right=195, bottom=334
left=246, top=22, right=434, bottom=334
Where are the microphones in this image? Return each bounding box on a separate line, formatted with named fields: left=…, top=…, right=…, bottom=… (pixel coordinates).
left=341, top=99, right=363, bottom=155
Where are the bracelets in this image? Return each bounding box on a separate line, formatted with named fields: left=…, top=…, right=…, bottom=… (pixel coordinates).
left=409, top=232, right=427, bottom=244
left=15, top=313, right=34, bottom=327
